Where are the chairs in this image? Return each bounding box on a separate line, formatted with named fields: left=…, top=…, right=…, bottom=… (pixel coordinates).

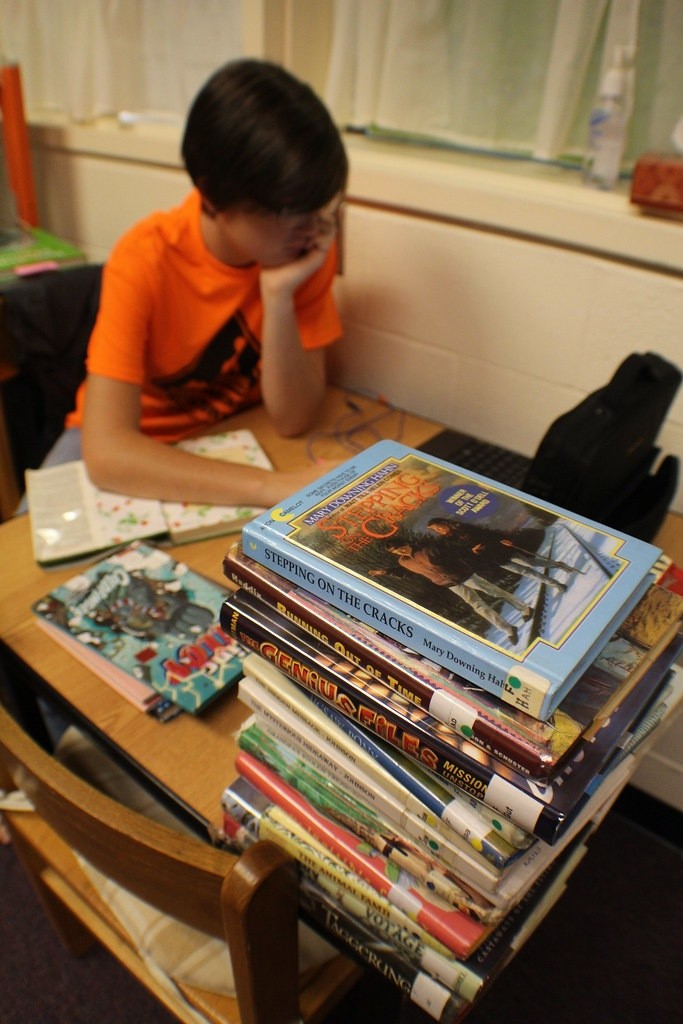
left=0, top=265, right=106, bottom=499
left=0, top=711, right=366, bottom=1024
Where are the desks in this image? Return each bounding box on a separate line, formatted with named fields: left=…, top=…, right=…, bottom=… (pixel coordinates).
left=0, top=391, right=683, bottom=1024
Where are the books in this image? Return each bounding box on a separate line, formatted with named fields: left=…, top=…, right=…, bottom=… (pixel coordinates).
left=29, top=540, right=249, bottom=723
left=0, top=222, right=85, bottom=291
left=220, top=440, right=683, bottom=1024
left=25, top=428, right=278, bottom=570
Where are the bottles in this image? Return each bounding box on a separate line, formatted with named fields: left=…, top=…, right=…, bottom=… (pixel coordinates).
left=579, top=43, right=638, bottom=188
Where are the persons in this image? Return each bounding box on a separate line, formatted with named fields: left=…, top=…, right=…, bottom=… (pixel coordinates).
left=19, top=58, right=348, bottom=507
left=384, top=539, right=533, bottom=644
left=427, top=518, right=571, bottom=592
